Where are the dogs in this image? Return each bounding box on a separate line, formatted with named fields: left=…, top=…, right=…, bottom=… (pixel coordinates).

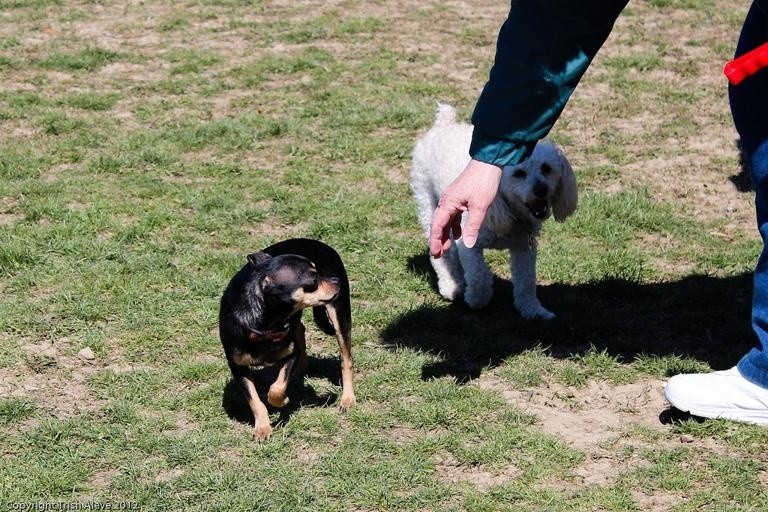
left=216, top=237, right=358, bottom=441
left=409, top=101, right=580, bottom=325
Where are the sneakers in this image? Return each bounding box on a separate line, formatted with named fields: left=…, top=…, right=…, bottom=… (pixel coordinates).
left=664, top=366, right=768, bottom=426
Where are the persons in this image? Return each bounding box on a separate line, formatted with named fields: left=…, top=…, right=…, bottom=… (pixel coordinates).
left=429, top=0, right=768, bottom=428
